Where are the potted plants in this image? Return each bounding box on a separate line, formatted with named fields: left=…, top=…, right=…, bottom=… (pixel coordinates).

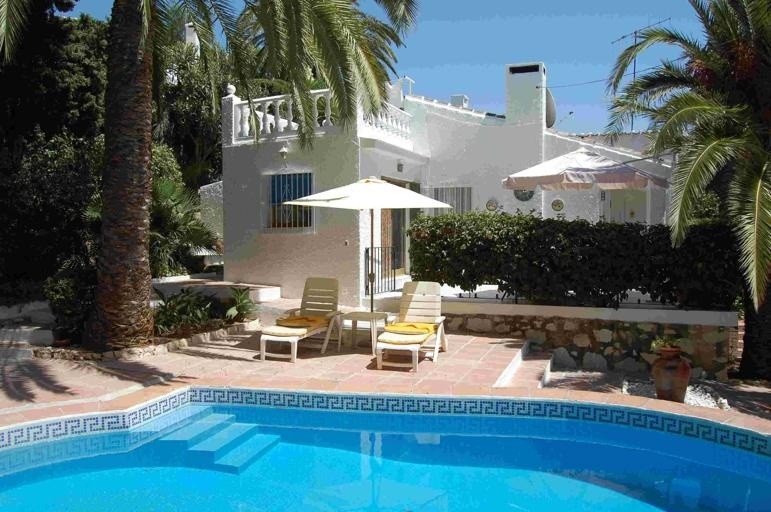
left=651, top=334, right=691, bottom=403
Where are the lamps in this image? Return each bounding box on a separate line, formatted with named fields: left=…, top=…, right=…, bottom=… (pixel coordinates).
left=279, top=144, right=287, bottom=159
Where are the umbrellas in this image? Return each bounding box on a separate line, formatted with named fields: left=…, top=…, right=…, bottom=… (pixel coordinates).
left=501, top=146, right=669, bottom=192
left=282, top=175, right=452, bottom=341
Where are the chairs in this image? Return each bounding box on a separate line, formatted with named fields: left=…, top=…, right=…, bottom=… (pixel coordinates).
left=376, top=279, right=447, bottom=370
left=259, top=277, right=341, bottom=360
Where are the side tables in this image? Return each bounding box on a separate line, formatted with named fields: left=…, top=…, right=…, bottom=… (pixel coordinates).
left=337, top=311, right=387, bottom=355
